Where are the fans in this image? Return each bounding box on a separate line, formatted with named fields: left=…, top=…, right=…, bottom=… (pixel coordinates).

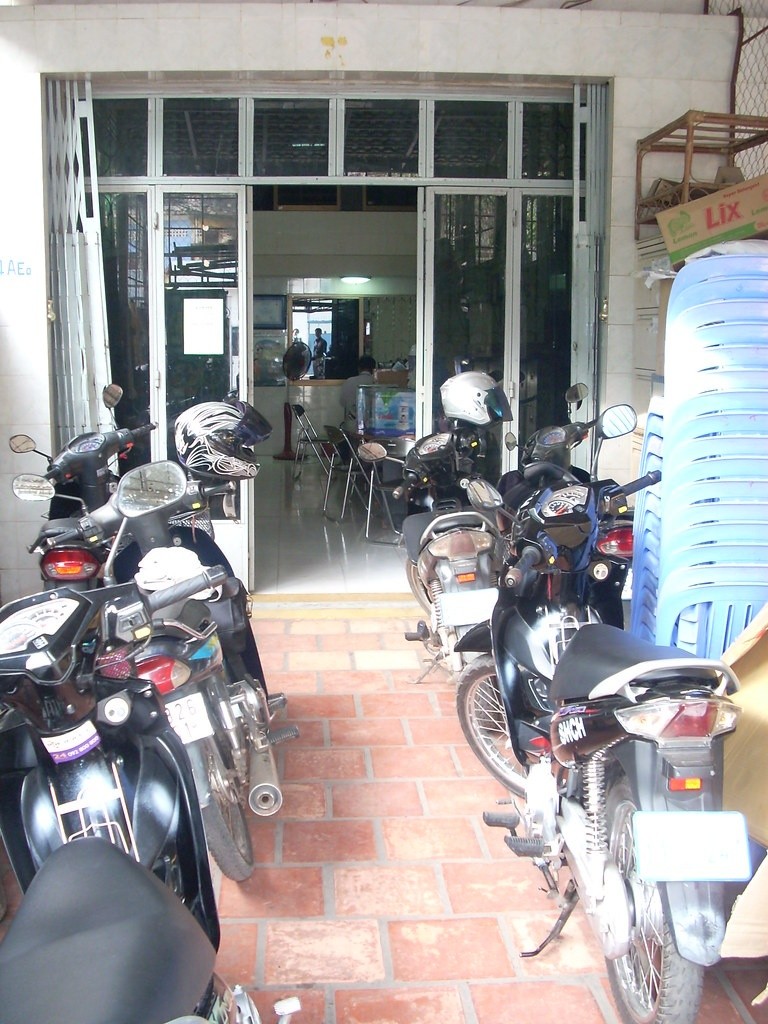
left=272, top=342, right=313, bottom=461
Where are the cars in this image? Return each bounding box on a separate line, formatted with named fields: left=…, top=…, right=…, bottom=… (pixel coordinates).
left=253, top=340, right=284, bottom=378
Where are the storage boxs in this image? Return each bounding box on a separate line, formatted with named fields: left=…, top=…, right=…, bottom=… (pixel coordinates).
left=713, top=166, right=745, bottom=185
left=712, top=601, right=768, bottom=849
left=648, top=178, right=675, bottom=201
left=653, top=169, right=768, bottom=274
left=373, top=368, right=409, bottom=390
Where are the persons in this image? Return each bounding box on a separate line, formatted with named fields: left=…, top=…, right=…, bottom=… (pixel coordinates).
left=292, top=328, right=301, bottom=342
left=311, top=328, right=327, bottom=379
left=334, top=354, right=375, bottom=470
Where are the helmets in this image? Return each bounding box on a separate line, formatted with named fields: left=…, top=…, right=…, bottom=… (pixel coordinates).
left=175, top=400, right=273, bottom=481
left=439, top=371, right=513, bottom=426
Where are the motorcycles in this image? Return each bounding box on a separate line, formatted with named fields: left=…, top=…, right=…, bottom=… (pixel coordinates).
left=496, top=383, right=635, bottom=630
left=453, top=402, right=754, bottom=1024
left=358, top=417, right=508, bottom=736
left=8, top=384, right=160, bottom=593
left=11, top=460, right=300, bottom=882
left=0, top=564, right=302, bottom=1024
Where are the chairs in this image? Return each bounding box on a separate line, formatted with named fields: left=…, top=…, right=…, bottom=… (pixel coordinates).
left=322, top=424, right=384, bottom=520
left=623, top=238, right=768, bottom=660
left=365, top=436, right=419, bottom=547
left=290, top=404, right=336, bottom=483
